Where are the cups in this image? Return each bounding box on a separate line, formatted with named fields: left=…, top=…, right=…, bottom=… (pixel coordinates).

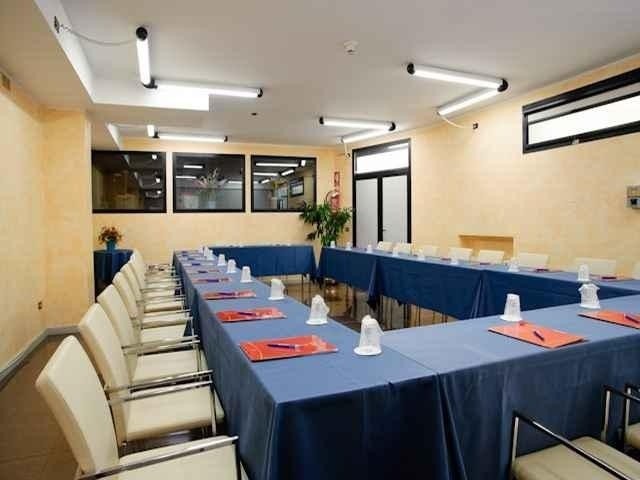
left=345, top=242, right=352, bottom=251
left=200, top=245, right=214, bottom=261
left=217, top=254, right=226, bottom=266
left=417, top=249, right=425, bottom=260
left=392, top=247, right=399, bottom=257
left=227, top=259, right=237, bottom=274
left=268, top=279, right=285, bottom=301
left=330, top=240, right=336, bottom=248
left=367, top=244, right=373, bottom=253
left=449, top=250, right=458, bottom=265
left=578, top=283, right=601, bottom=309
left=507, top=256, right=519, bottom=272
left=500, top=293, right=523, bottom=322
left=306, top=294, right=330, bottom=325
left=354, top=315, right=384, bottom=356
left=576, top=264, right=590, bottom=282
left=240, top=266, right=252, bottom=283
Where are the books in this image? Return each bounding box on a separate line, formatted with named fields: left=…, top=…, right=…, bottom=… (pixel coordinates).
left=577, top=310, right=640, bottom=330
left=176, top=245, right=257, bottom=304
left=491, top=320, right=585, bottom=351
left=240, top=333, right=340, bottom=362
left=212, top=305, right=291, bottom=326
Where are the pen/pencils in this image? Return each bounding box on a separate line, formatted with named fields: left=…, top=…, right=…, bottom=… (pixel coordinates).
left=238, top=312, right=256, bottom=316
left=534, top=330, right=544, bottom=340
left=206, top=280, right=219, bottom=282
left=536, top=269, right=549, bottom=271
left=218, top=292, right=235, bottom=295
left=480, top=262, right=491, bottom=264
left=602, top=277, right=618, bottom=280
left=198, top=271, right=208, bottom=273
left=191, top=264, right=200, bottom=265
left=268, top=344, right=305, bottom=348
left=625, top=315, right=640, bottom=323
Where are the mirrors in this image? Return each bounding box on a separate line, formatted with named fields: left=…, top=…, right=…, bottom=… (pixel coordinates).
left=93, top=149, right=168, bottom=215
left=250, top=154, right=318, bottom=213
left=172, top=151, right=246, bottom=214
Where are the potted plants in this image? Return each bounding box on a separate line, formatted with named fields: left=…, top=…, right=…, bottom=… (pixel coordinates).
left=97, top=224, right=123, bottom=251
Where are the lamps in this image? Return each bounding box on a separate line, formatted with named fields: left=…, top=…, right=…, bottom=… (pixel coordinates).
left=150, top=152, right=163, bottom=195
left=145, top=125, right=229, bottom=145
left=184, top=164, right=204, bottom=170
left=253, top=160, right=306, bottom=185
left=319, top=115, right=397, bottom=144
left=135, top=26, right=265, bottom=100
left=176, top=174, right=197, bottom=179
left=407, top=63, right=509, bottom=117
